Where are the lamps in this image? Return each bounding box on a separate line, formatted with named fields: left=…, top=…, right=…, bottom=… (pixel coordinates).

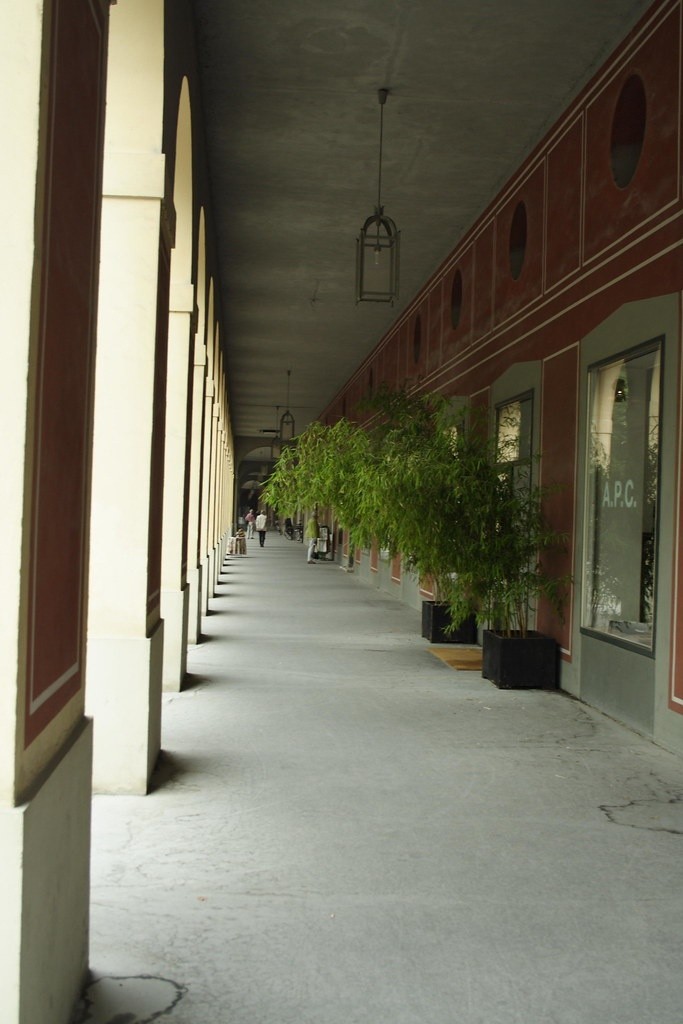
left=258, top=368, right=298, bottom=479
left=349, top=86, right=404, bottom=311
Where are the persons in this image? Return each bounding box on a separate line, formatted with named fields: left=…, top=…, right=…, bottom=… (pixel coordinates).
left=245, top=509, right=256, bottom=539
left=305, top=511, right=319, bottom=564
left=256, top=510, right=267, bottom=547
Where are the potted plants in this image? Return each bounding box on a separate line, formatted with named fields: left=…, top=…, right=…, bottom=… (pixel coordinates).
left=255, top=371, right=480, bottom=647
left=308, top=393, right=577, bottom=692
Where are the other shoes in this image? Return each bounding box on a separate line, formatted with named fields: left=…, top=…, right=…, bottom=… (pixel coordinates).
left=308, top=561, right=316, bottom=564
